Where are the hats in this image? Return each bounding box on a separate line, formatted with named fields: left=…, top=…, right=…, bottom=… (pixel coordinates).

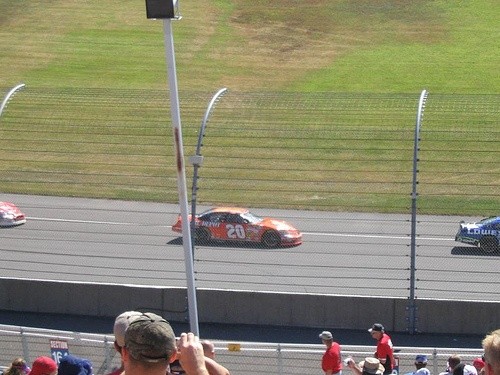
left=463, top=365, right=477, bottom=375
left=114, top=311, right=142, bottom=347
left=415, top=368, right=430, bottom=375
left=358, top=357, right=385, bottom=375
left=28, top=356, right=57, bottom=375
left=415, top=354, right=428, bottom=365
left=473, top=358, right=486, bottom=368
left=125, top=312, right=176, bottom=363
left=368, top=323, right=384, bottom=333
left=57, top=353, right=92, bottom=375
left=319, top=331, right=332, bottom=340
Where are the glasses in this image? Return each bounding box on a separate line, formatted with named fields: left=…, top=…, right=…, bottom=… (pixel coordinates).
left=481, top=353, right=490, bottom=366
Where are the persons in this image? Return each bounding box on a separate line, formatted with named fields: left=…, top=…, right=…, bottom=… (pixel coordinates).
left=318, top=331, right=343, bottom=375
left=367, top=323, right=396, bottom=374
left=344, top=330, right=500, bottom=375
left=2, top=310, right=230, bottom=375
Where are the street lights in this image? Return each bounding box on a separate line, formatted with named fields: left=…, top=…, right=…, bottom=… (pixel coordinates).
left=145, top=1, right=199, bottom=338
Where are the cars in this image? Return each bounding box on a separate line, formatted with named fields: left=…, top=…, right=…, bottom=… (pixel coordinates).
left=0, top=201, right=26, bottom=228
left=454, top=215, right=500, bottom=253
left=173, top=205, right=304, bottom=249
